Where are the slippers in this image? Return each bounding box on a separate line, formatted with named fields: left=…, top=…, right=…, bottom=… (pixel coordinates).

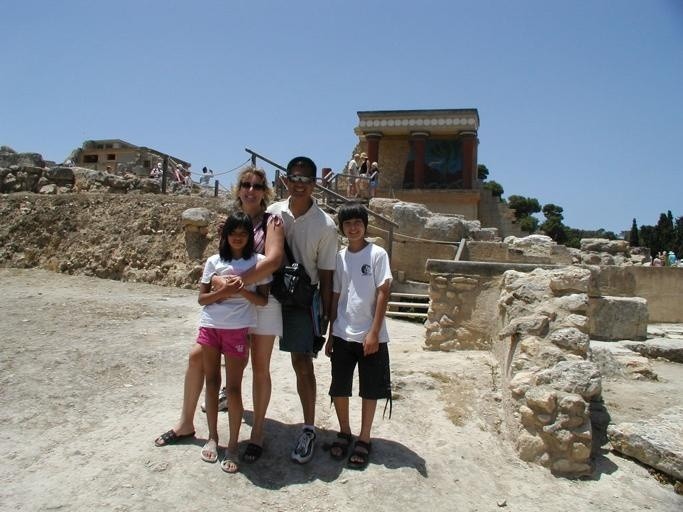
left=155, top=430, right=195, bottom=447
left=201, top=442, right=263, bottom=472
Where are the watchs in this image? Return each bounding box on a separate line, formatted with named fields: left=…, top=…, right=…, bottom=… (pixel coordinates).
left=321, top=314, right=329, bottom=320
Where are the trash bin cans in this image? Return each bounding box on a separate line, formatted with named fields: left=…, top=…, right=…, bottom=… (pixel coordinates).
left=322, top=168, right=332, bottom=187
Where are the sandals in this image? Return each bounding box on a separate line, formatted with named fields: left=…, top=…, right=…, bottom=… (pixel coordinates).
left=330, top=432, right=371, bottom=468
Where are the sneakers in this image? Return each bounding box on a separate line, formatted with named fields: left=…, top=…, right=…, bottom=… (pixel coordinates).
left=201, top=387, right=228, bottom=412
left=291, top=428, right=317, bottom=464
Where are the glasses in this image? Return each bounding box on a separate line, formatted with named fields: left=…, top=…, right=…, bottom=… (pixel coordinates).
left=287, top=176, right=313, bottom=184
left=241, top=182, right=265, bottom=190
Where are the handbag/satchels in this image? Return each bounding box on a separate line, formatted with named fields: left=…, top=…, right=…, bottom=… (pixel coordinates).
left=270, top=263, right=311, bottom=309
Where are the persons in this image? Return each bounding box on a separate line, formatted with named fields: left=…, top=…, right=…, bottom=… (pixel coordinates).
left=365, top=162, right=379, bottom=198
left=183, top=170, right=194, bottom=188
left=198, top=210, right=273, bottom=475
left=101, top=166, right=115, bottom=187
left=324, top=199, right=394, bottom=473
left=652, top=255, right=660, bottom=267
left=173, top=163, right=185, bottom=183
left=657, top=250, right=667, bottom=267
left=200, top=155, right=338, bottom=468
left=666, top=251, right=676, bottom=266
left=199, top=166, right=215, bottom=188
left=345, top=153, right=360, bottom=197
left=154, top=165, right=287, bottom=468
left=149, top=163, right=163, bottom=181
left=354, top=152, right=370, bottom=197
